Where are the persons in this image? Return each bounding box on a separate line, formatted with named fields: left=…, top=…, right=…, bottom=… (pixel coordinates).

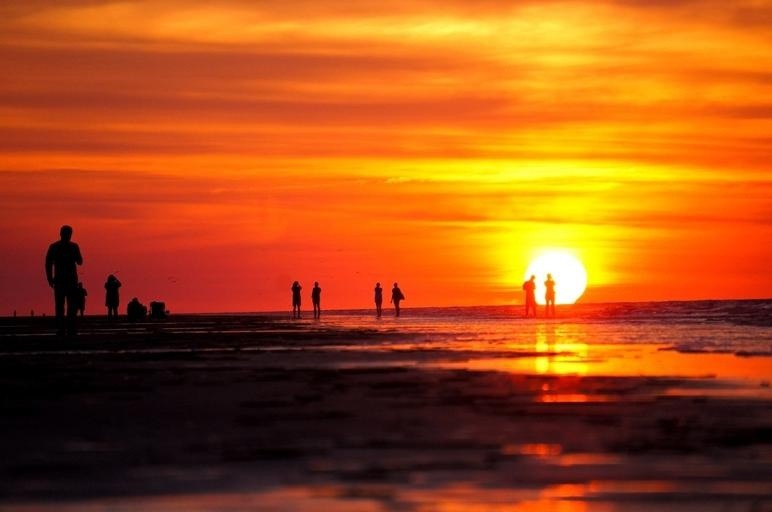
left=373, top=282, right=383, bottom=317
left=104, top=274, right=121, bottom=315
left=544, top=273, right=556, bottom=316
left=290, top=281, right=302, bottom=317
left=523, top=275, right=537, bottom=317
left=127, top=297, right=147, bottom=315
left=44, top=223, right=83, bottom=341
left=78, top=282, right=87, bottom=316
left=312, top=281, right=321, bottom=318
left=390, top=282, right=404, bottom=317
